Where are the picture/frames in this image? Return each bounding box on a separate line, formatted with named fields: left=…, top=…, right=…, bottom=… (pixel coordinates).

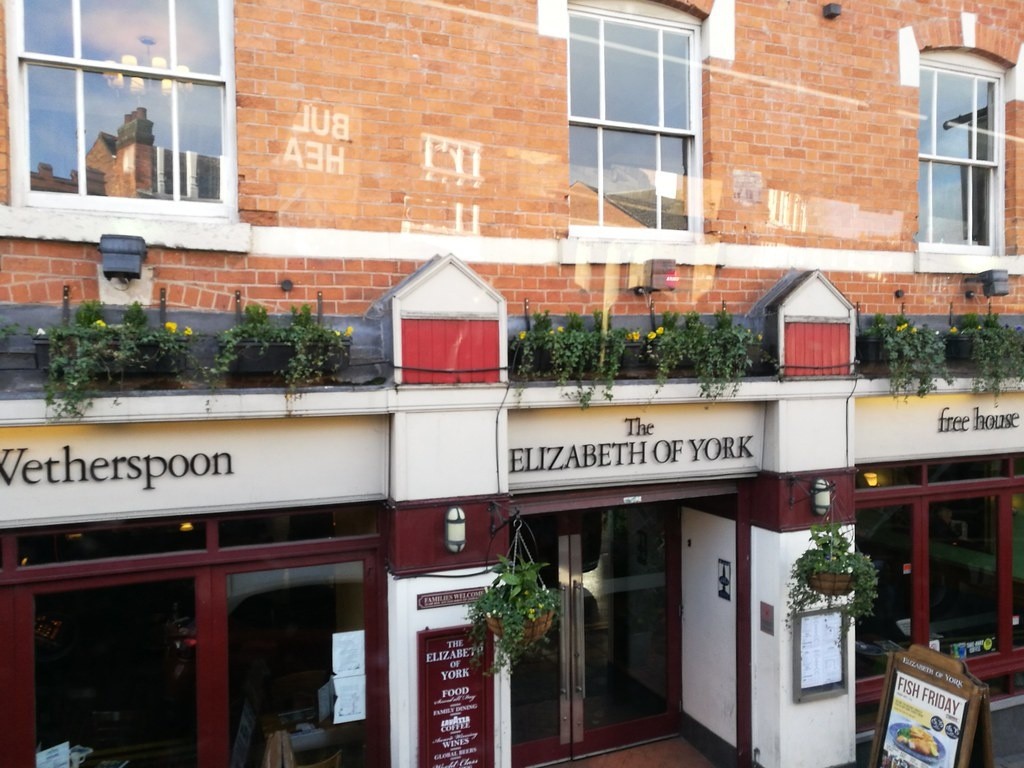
left=889, top=460, right=1002, bottom=555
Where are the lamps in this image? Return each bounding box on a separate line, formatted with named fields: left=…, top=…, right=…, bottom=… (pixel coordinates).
left=965, top=268, right=1010, bottom=298
left=635, top=259, right=677, bottom=295
left=442, top=505, right=468, bottom=553
left=97, top=233, right=148, bottom=289
left=810, top=477, right=833, bottom=517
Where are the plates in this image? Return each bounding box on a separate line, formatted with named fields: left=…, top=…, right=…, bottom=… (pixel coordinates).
left=890, top=724, right=945, bottom=762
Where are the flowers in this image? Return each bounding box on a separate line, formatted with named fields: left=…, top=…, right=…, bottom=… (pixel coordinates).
left=465, top=554, right=564, bottom=678
left=647, top=308, right=786, bottom=405
left=511, top=309, right=643, bottom=410
left=864, top=312, right=957, bottom=401
left=948, top=311, right=1024, bottom=408
left=781, top=519, right=879, bottom=650
left=28, top=297, right=214, bottom=427
left=207, top=301, right=355, bottom=416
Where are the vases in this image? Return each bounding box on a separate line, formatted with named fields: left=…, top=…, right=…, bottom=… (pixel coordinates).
left=646, top=336, right=764, bottom=369
left=32, top=334, right=195, bottom=377
left=221, top=339, right=352, bottom=374
left=859, top=333, right=946, bottom=367
left=805, top=570, right=857, bottom=596
left=517, top=340, right=643, bottom=373
left=486, top=611, right=555, bottom=644
left=946, top=333, right=991, bottom=361
